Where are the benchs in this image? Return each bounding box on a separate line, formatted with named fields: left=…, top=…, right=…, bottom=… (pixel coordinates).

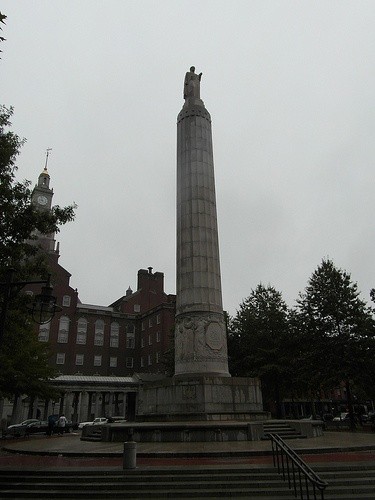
left=1, top=425, right=30, bottom=440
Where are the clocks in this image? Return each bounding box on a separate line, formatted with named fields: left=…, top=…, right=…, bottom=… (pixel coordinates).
left=37, top=195, right=47, bottom=206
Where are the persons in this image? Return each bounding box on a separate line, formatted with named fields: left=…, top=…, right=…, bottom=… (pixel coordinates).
left=47, top=413, right=68, bottom=438
left=344, top=403, right=366, bottom=430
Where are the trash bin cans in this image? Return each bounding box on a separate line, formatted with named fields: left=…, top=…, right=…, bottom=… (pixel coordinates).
left=123, top=442, right=137, bottom=469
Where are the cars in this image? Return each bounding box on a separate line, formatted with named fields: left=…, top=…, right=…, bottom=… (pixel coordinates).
left=300, top=410, right=375, bottom=426
left=7, top=415, right=128, bottom=436
